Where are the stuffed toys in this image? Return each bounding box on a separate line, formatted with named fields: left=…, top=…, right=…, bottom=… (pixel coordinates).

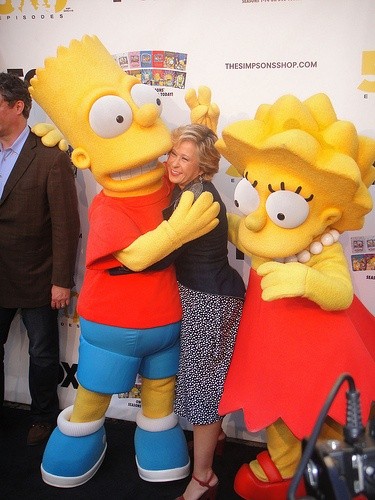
left=28, top=35, right=220, bottom=487
left=214, top=93, right=374, bottom=496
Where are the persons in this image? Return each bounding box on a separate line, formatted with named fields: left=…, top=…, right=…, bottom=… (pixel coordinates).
left=0, top=76, right=79, bottom=446
left=166, top=126, right=245, bottom=500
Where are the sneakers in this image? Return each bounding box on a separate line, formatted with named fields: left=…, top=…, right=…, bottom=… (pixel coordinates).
left=27, top=420, right=57, bottom=446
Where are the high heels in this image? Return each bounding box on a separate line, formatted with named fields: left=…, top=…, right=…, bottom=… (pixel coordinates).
left=188, top=429, right=226, bottom=456
left=175, top=471, right=220, bottom=500
